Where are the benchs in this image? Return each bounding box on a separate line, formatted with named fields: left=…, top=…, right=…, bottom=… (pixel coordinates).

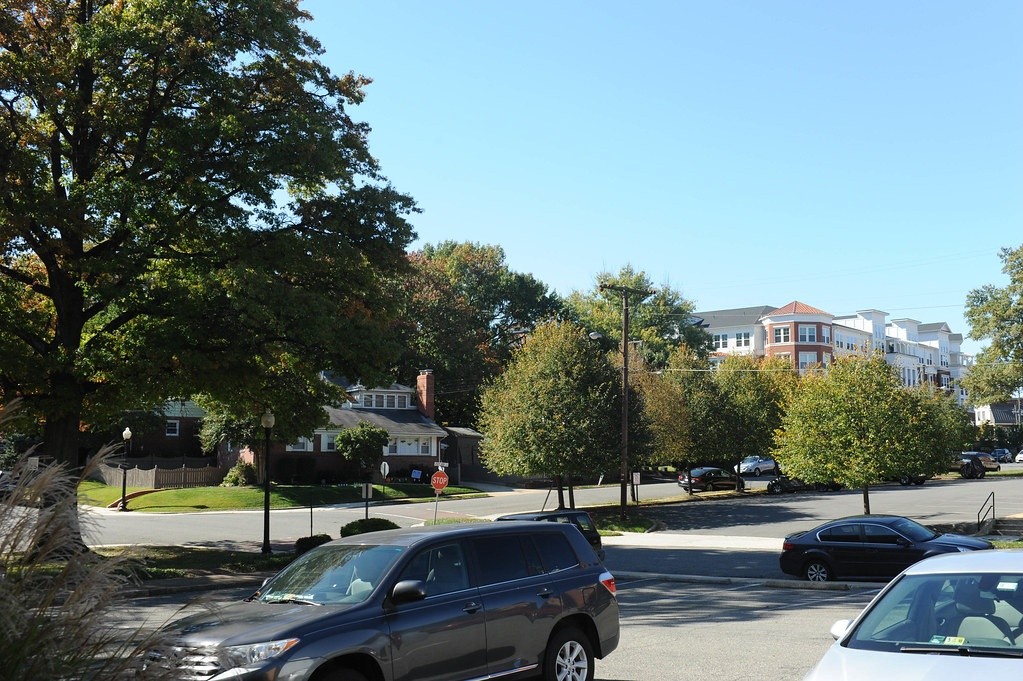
left=992, top=589, right=1023, bottom=648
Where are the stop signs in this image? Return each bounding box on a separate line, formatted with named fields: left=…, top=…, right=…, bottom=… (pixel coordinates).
left=432, top=472, right=448, bottom=491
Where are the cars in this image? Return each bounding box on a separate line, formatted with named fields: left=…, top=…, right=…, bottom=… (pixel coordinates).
left=494, top=510, right=606, bottom=565
left=801, top=548, right=1022, bottom=681
left=879, top=469, right=933, bottom=487
left=1014, top=451, right=1023, bottom=464
left=779, top=514, right=998, bottom=582
left=766, top=472, right=841, bottom=495
left=678, top=467, right=746, bottom=494
left=947, top=451, right=1001, bottom=471
left=991, top=449, right=1013, bottom=464
left=734, top=455, right=776, bottom=479
left=116, top=523, right=620, bottom=680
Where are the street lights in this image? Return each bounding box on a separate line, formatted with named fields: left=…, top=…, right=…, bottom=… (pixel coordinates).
left=118, top=427, right=132, bottom=514
left=589, top=330, right=628, bottom=521
left=261, top=406, right=275, bottom=555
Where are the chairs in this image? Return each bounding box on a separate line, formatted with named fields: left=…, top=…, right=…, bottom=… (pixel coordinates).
left=941, top=584, right=1015, bottom=645
left=344, top=558, right=381, bottom=595
left=425, top=550, right=463, bottom=595
